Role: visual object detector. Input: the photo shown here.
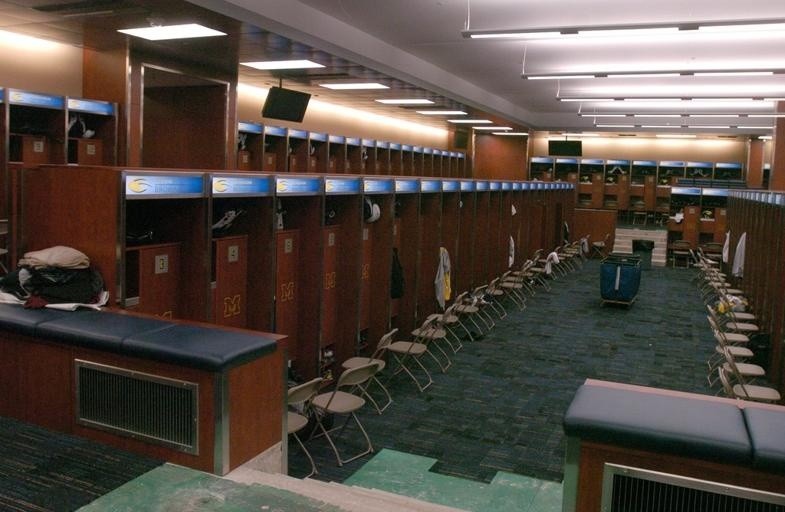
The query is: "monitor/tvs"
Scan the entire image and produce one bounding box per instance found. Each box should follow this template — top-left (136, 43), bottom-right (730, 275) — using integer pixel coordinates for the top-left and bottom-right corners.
top-left (549, 141), bottom-right (583, 157)
top-left (261, 87), bottom-right (312, 122)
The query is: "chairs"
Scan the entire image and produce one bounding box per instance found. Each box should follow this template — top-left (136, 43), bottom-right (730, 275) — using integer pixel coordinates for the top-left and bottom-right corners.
top-left (672, 237), bottom-right (781, 407)
